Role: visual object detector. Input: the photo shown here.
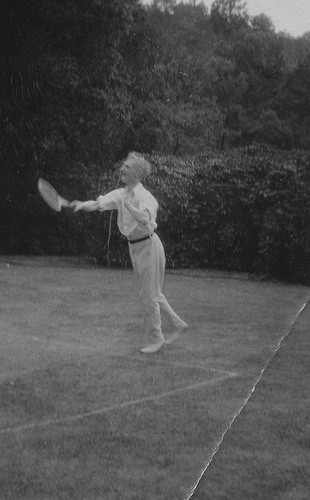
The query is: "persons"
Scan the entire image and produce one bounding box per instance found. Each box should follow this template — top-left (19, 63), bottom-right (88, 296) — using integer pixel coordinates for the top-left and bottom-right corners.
top-left (70, 151), bottom-right (189, 354)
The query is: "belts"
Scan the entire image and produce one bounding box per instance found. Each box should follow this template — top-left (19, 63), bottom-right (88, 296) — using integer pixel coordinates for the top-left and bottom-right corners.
top-left (128, 231), bottom-right (155, 244)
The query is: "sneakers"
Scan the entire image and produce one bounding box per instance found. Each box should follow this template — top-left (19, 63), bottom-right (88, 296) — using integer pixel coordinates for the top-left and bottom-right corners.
top-left (164, 322), bottom-right (189, 345)
top-left (140, 338), bottom-right (165, 353)
